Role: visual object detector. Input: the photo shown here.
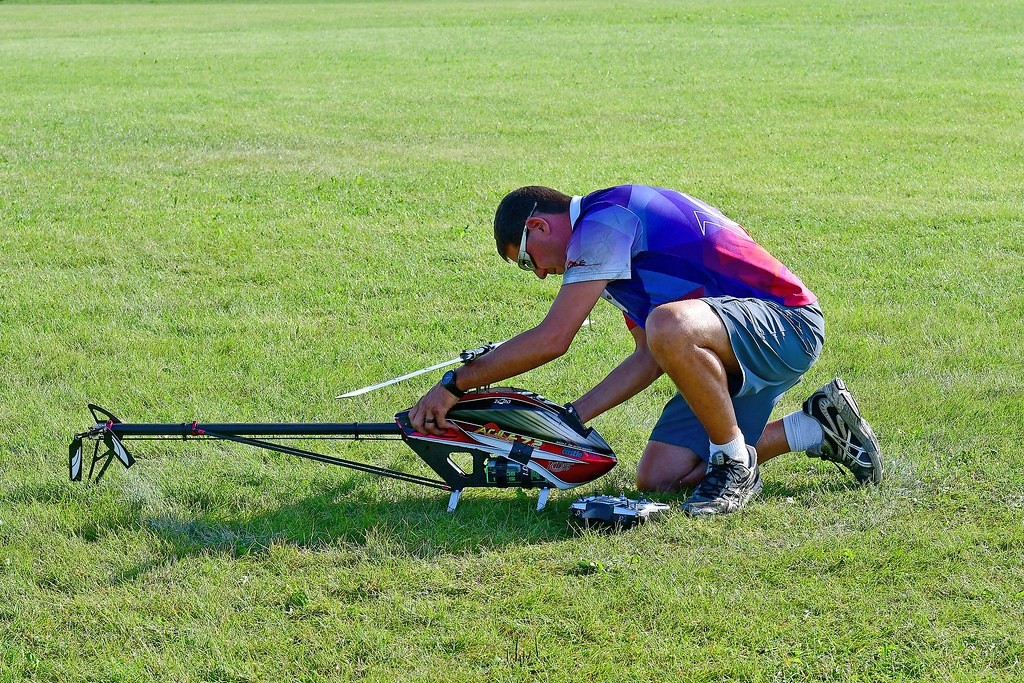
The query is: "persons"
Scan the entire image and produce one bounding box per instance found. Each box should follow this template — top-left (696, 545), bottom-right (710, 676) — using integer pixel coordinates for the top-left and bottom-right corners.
top-left (409, 185), bottom-right (884, 518)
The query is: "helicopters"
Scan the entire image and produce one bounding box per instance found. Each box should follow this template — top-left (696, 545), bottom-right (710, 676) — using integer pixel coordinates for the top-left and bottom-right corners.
top-left (69, 320), bottom-right (618, 513)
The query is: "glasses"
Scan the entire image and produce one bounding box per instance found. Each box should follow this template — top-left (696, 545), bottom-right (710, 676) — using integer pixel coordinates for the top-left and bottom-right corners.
top-left (517, 201), bottom-right (538, 271)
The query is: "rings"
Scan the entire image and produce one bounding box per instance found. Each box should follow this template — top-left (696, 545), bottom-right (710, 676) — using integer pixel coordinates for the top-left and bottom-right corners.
top-left (425, 418), bottom-right (435, 423)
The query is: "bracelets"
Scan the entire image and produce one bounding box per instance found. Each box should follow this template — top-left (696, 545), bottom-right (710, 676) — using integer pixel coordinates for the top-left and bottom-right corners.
top-left (563, 402), bottom-right (583, 425)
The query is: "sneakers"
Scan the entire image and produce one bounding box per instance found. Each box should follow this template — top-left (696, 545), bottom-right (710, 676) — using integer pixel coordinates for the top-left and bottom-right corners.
top-left (802, 376), bottom-right (884, 486)
top-left (681, 443), bottom-right (763, 518)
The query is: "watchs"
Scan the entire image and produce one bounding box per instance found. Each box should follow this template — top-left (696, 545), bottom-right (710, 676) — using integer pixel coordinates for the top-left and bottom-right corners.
top-left (441, 370), bottom-right (465, 398)
